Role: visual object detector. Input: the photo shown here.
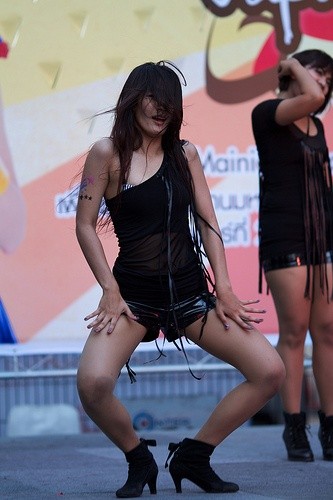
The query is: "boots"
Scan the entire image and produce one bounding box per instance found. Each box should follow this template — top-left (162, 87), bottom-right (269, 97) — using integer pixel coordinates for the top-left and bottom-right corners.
top-left (166, 437), bottom-right (239, 494)
top-left (116, 438), bottom-right (157, 497)
top-left (282, 410), bottom-right (316, 462)
top-left (318, 410), bottom-right (333, 461)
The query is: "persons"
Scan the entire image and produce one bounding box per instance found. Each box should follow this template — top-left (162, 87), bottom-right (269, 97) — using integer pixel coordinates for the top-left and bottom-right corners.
top-left (250, 49), bottom-right (333, 461)
top-left (74, 62), bottom-right (287, 497)
